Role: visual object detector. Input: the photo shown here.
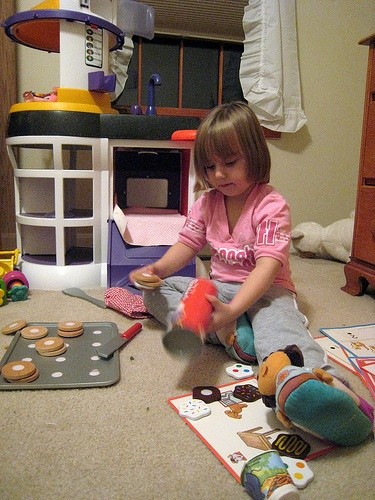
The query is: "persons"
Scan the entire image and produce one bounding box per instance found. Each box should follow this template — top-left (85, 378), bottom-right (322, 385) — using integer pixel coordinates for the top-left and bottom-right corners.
top-left (130, 102), bottom-right (375, 447)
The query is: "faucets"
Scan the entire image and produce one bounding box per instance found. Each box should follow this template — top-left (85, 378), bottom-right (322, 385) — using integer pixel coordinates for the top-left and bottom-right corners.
top-left (146, 73), bottom-right (163, 116)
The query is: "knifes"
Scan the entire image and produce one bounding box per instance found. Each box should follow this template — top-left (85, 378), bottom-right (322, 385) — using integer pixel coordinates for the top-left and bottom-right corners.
top-left (96, 323), bottom-right (142, 357)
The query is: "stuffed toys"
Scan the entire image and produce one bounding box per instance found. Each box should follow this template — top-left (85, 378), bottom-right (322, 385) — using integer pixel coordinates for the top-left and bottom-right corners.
top-left (258, 343), bottom-right (333, 429)
top-left (290, 212), bottom-right (355, 262)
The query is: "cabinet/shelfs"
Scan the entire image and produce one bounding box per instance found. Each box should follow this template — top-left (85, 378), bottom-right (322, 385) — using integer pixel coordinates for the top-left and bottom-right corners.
top-left (5, 102), bottom-right (201, 297)
top-left (340, 34), bottom-right (375, 300)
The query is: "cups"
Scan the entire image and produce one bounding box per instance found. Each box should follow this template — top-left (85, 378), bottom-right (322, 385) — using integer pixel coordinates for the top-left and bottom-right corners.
top-left (3, 270), bottom-right (30, 293)
